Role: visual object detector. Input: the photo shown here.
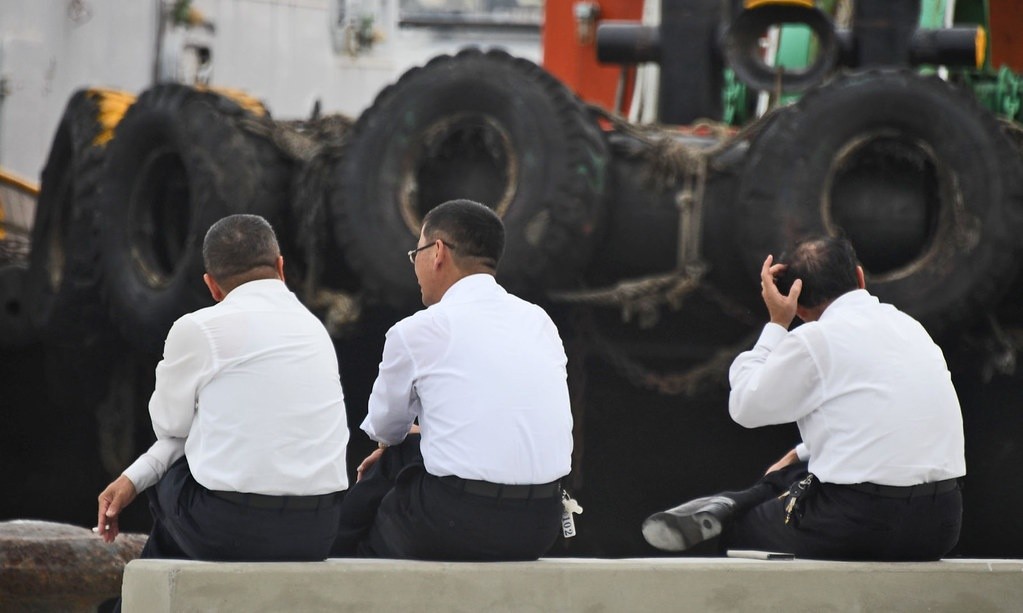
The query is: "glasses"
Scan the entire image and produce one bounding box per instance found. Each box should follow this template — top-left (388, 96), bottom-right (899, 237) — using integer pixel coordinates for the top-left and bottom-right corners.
top-left (407, 241), bottom-right (455, 264)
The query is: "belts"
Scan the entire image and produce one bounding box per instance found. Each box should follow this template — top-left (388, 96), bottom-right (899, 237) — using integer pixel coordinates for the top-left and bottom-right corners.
top-left (434, 475), bottom-right (562, 501)
top-left (202, 485), bottom-right (335, 513)
top-left (840, 477), bottom-right (960, 499)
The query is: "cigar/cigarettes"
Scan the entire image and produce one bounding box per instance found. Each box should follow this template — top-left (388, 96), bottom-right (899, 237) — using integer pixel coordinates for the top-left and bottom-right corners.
top-left (92, 525), bottom-right (109, 533)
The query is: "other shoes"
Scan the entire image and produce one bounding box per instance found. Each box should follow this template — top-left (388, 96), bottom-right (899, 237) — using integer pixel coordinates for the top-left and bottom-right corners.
top-left (96, 596), bottom-right (121, 613)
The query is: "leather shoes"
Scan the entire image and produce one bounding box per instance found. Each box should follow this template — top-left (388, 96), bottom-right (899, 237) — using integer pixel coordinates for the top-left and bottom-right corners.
top-left (640, 496), bottom-right (736, 552)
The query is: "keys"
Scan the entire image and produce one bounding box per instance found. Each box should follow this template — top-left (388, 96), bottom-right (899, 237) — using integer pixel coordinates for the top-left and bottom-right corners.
top-left (777, 480), bottom-right (808, 525)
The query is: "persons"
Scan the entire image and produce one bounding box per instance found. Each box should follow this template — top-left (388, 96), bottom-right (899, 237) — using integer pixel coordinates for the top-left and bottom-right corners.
top-left (642, 228), bottom-right (966, 561)
top-left (329, 199), bottom-right (575, 562)
top-left (97, 215), bottom-right (350, 613)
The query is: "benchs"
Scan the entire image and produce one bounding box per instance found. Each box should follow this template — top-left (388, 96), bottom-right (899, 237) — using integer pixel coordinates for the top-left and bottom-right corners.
top-left (119, 556), bottom-right (1023, 613)
